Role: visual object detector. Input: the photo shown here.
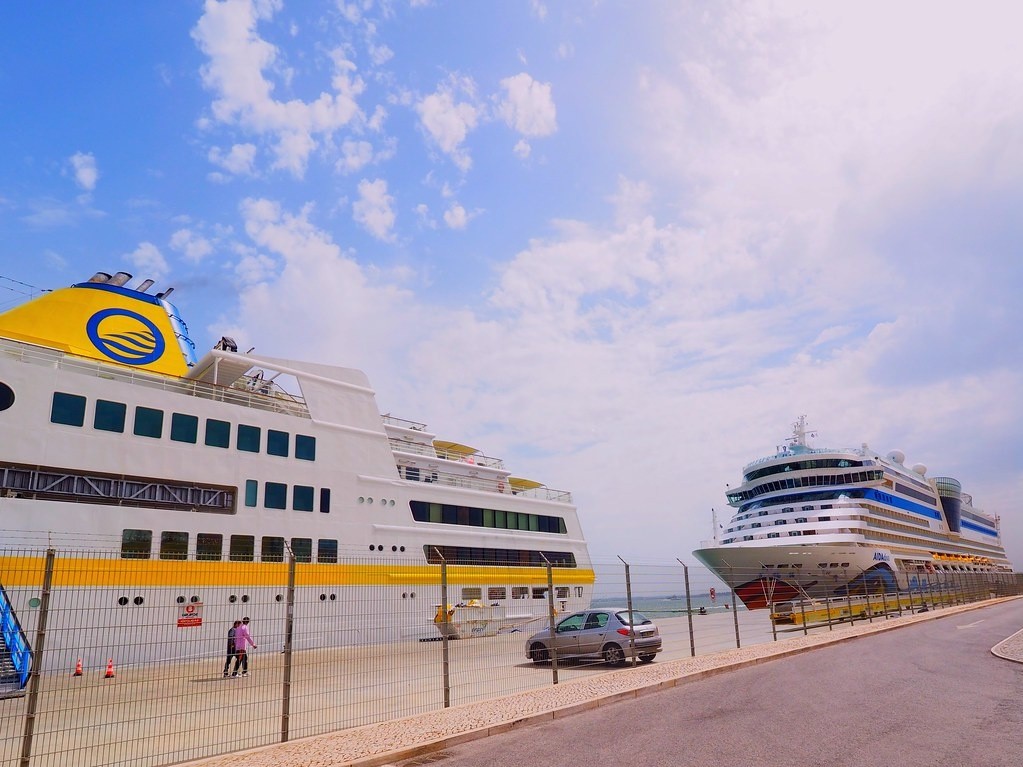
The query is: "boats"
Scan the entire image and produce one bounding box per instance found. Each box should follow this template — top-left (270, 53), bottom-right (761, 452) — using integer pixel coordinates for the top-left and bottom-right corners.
top-left (692, 414), bottom-right (1017, 614)
top-left (1, 271), bottom-right (596, 677)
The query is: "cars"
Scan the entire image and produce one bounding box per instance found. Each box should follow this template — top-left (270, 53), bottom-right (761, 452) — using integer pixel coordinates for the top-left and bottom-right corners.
top-left (525, 607), bottom-right (663, 668)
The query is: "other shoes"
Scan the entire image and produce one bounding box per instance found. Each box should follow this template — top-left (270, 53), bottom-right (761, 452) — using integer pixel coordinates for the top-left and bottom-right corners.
top-left (242, 672), bottom-right (250, 677)
top-left (231, 673), bottom-right (242, 679)
top-left (224, 675), bottom-right (230, 678)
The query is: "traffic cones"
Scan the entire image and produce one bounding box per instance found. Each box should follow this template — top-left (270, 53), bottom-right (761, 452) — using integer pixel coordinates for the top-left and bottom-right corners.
top-left (73, 658), bottom-right (84, 676)
top-left (105, 659), bottom-right (115, 678)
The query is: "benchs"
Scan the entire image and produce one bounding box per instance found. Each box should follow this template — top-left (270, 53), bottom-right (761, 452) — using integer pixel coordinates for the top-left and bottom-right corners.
top-left (822, 611), bottom-right (867, 623)
top-left (873, 606), bottom-right (902, 615)
top-left (906, 602), bottom-right (927, 609)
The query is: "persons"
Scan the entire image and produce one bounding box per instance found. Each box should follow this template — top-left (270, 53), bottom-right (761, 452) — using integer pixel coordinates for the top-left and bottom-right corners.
top-left (230, 617), bottom-right (258, 679)
top-left (699, 606), bottom-right (706, 613)
top-left (223, 620), bottom-right (241, 677)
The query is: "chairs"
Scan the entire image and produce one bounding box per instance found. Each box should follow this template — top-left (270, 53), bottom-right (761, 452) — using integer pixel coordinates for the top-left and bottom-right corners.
top-left (591, 617), bottom-right (601, 628)
